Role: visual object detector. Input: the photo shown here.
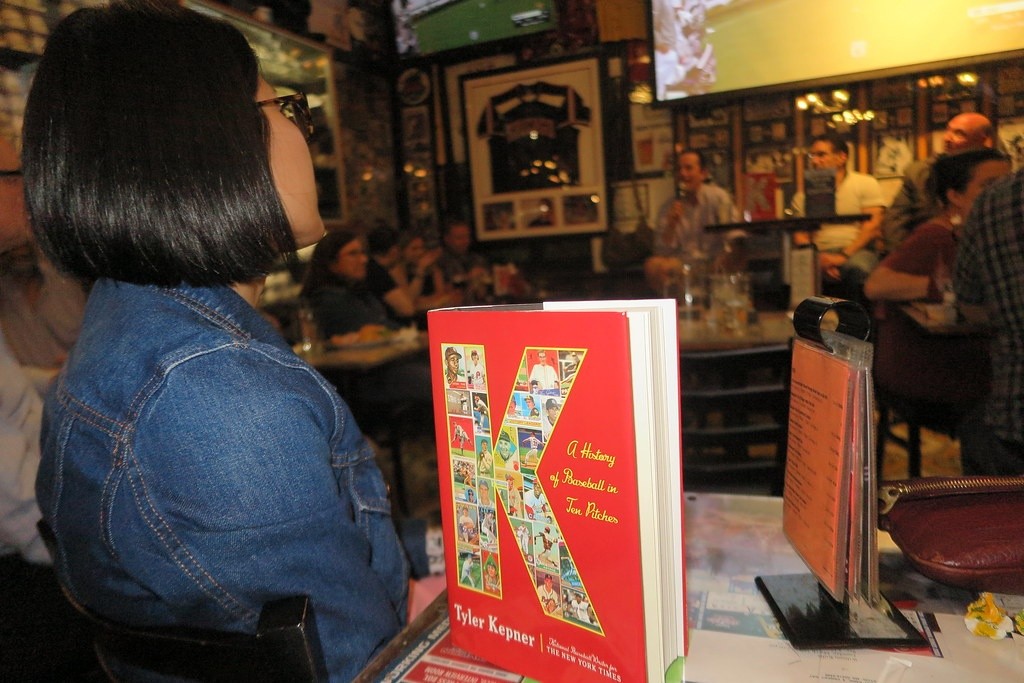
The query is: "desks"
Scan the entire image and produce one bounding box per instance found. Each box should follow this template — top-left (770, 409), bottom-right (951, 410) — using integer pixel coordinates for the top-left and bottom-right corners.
top-left (291, 331), bottom-right (430, 518)
top-left (672, 301), bottom-right (836, 458)
top-left (351, 489), bottom-right (1024, 683)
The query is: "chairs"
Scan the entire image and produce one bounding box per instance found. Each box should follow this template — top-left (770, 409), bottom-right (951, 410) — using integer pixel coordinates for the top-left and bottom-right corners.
top-left (673, 338), bottom-right (793, 495)
top-left (34, 518), bottom-right (329, 683)
top-left (839, 271), bottom-right (921, 483)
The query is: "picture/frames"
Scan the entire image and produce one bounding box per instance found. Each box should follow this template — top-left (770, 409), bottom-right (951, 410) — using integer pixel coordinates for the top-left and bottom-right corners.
top-left (458, 47), bottom-right (613, 247)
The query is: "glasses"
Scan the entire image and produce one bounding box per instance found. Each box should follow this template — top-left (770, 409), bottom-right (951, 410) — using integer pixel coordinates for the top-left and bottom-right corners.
top-left (255, 92), bottom-right (315, 146)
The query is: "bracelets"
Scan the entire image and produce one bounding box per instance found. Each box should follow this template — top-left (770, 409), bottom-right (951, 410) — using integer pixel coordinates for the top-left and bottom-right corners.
top-left (839, 250), bottom-right (848, 258)
top-left (927, 275), bottom-right (939, 301)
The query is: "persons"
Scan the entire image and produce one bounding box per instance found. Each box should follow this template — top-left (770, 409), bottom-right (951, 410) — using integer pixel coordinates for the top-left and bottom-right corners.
top-left (862, 113), bottom-right (1024, 480)
top-left (793, 133), bottom-right (882, 291)
top-left (646, 148), bottom-right (745, 294)
top-left (303, 220), bottom-right (490, 408)
top-left (1, 172), bottom-right (84, 573)
top-left (443, 346), bottom-right (598, 626)
top-left (21, 0), bottom-right (443, 683)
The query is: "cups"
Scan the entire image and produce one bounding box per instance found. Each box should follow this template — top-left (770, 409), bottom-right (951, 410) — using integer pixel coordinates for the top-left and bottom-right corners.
top-left (725, 300), bottom-right (745, 339)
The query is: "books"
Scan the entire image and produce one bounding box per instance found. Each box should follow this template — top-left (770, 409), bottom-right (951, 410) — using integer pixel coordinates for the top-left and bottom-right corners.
top-left (429, 297), bottom-right (689, 683)
top-left (740, 172), bottom-right (783, 220)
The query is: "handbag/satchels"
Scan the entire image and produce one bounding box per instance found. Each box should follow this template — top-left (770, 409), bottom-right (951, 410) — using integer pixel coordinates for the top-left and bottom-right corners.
top-left (876, 475), bottom-right (1024, 593)
